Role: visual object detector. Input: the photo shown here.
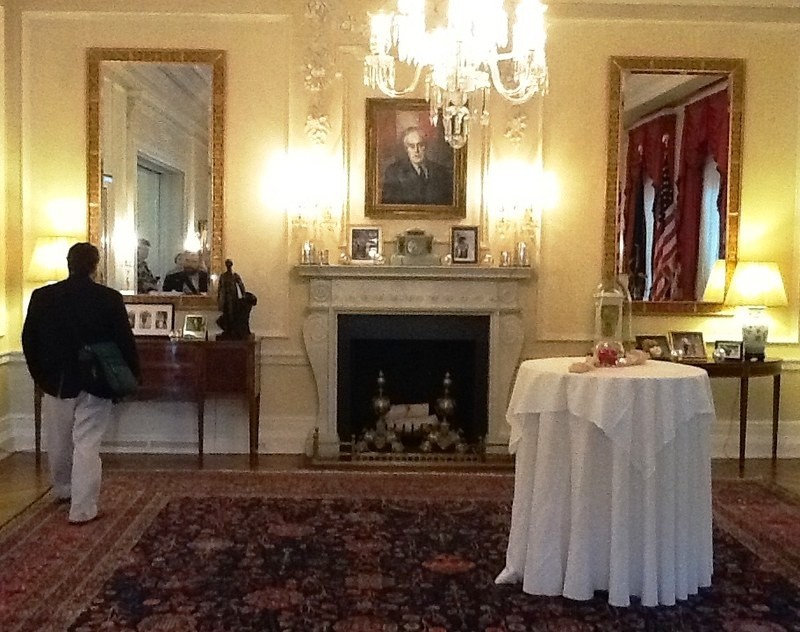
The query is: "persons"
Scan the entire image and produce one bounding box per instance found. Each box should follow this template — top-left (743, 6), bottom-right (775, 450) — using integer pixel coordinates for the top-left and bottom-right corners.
top-left (216, 258), bottom-right (245, 337)
top-left (21, 242), bottom-right (144, 525)
top-left (137, 238), bottom-right (208, 294)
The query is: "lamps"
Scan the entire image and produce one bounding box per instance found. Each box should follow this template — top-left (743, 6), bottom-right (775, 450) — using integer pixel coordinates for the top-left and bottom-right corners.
top-left (723, 260), bottom-right (788, 361)
top-left (363, 0), bottom-right (550, 146)
top-left (703, 256), bottom-right (725, 303)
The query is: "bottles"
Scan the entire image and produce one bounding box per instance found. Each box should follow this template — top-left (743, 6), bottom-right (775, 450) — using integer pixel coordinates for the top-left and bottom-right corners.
top-left (713, 346), bottom-right (726, 364)
top-left (595, 337), bottom-right (624, 366)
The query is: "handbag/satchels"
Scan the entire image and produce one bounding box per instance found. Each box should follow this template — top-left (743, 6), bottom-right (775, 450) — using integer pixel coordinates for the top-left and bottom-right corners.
top-left (84, 340), bottom-right (139, 395)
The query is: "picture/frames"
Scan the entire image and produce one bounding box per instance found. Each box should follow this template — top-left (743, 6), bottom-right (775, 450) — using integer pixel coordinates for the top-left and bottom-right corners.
top-left (126, 306), bottom-right (175, 334)
top-left (666, 328), bottom-right (708, 359)
top-left (348, 226), bottom-right (383, 265)
top-left (365, 97), bottom-right (466, 220)
top-left (449, 225), bottom-right (478, 264)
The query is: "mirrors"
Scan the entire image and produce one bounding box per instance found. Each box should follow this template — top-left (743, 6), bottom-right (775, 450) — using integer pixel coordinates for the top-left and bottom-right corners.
top-left (607, 53), bottom-right (746, 321)
top-left (85, 46), bottom-right (227, 309)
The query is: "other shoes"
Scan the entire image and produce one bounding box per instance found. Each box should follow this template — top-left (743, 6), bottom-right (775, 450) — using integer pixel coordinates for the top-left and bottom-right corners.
top-left (95, 511), bottom-right (106, 518)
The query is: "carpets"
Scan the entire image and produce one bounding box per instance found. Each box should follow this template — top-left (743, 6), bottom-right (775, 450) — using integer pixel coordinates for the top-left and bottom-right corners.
top-left (0, 470), bottom-right (799, 632)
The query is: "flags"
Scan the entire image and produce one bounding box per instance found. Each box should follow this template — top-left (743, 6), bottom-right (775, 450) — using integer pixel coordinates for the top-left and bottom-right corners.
top-left (623, 134), bottom-right (681, 300)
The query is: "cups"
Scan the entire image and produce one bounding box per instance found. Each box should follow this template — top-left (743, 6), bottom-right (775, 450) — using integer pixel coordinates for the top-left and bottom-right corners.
top-left (670, 349), bottom-right (683, 361)
top-left (516, 242), bottom-right (526, 267)
top-left (500, 251), bottom-right (512, 266)
top-left (302, 243), bottom-right (314, 264)
top-left (318, 250), bottom-right (329, 265)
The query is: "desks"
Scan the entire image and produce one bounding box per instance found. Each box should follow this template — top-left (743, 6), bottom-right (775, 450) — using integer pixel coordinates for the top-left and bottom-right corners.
top-left (658, 359), bottom-right (783, 469)
top-left (33, 334), bottom-right (265, 475)
top-left (495, 357), bottom-right (718, 606)
